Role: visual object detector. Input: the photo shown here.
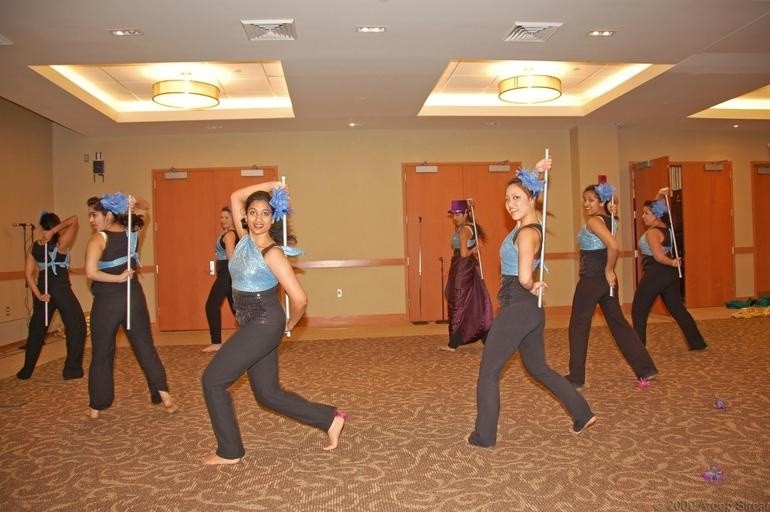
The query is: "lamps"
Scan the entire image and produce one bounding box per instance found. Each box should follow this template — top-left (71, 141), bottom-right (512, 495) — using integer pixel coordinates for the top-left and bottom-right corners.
top-left (149, 80), bottom-right (224, 110)
top-left (497, 77), bottom-right (564, 106)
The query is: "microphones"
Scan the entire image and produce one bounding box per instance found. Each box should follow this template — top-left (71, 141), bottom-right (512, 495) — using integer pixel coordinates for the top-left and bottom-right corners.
top-left (13, 223), bottom-right (32, 226)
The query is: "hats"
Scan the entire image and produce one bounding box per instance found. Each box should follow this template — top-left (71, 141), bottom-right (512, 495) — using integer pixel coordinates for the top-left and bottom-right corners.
top-left (448, 199), bottom-right (473, 214)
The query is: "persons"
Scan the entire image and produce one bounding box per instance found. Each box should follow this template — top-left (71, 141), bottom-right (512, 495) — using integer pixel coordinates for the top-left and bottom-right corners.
top-left (14, 180), bottom-right (346, 466)
top-left (439, 157), bottom-right (708, 450)
